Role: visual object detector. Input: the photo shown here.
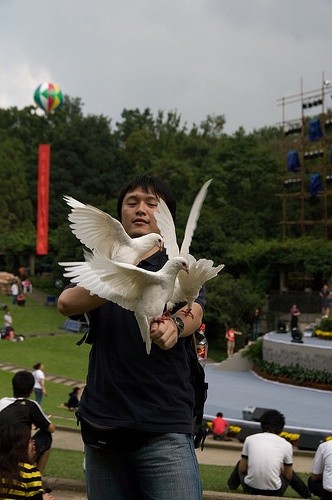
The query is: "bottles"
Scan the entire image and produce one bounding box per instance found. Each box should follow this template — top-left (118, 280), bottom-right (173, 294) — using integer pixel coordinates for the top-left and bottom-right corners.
top-left (196, 323), bottom-right (208, 368)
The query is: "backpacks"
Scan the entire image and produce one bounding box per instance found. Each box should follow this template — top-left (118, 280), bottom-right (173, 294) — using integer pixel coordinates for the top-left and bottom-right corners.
top-left (173, 300), bottom-right (208, 451)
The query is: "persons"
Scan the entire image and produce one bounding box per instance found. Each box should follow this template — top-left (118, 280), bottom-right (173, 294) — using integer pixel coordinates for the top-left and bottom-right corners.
top-left (61, 386), bottom-right (80, 411)
top-left (0, 371), bottom-right (55, 494)
top-left (225, 326), bottom-right (243, 357)
top-left (319, 285), bottom-right (332, 318)
top-left (290, 304), bottom-right (301, 329)
top-left (32, 363), bottom-right (47, 405)
top-left (227, 409), bottom-right (313, 499)
top-left (212, 412), bottom-right (232, 442)
top-left (250, 307), bottom-right (260, 340)
top-left (10, 280), bottom-right (20, 304)
top-left (0, 423), bottom-right (56, 500)
top-left (4, 309), bottom-right (13, 337)
top-left (307, 439), bottom-right (332, 500)
top-left (57, 176), bottom-right (207, 500)
top-left (22, 278), bottom-right (32, 294)
top-left (16, 293), bottom-right (27, 306)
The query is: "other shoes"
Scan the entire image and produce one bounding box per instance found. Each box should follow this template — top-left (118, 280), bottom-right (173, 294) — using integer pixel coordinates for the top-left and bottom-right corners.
top-left (227, 485), bottom-right (238, 492)
top-left (41, 482), bottom-right (52, 493)
top-left (308, 492), bottom-right (314, 499)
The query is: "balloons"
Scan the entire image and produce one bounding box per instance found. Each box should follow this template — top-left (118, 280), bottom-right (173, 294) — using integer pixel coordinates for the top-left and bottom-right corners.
top-left (33, 82), bottom-right (64, 112)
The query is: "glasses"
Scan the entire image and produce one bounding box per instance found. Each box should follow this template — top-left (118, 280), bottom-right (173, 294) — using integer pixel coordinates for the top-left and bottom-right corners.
top-left (30, 438), bottom-right (35, 444)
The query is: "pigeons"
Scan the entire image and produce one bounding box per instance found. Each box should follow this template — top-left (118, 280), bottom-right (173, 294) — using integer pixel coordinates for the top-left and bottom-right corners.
top-left (58, 178), bottom-right (225, 354)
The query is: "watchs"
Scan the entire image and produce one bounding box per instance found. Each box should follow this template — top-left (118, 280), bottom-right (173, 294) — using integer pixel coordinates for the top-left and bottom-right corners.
top-left (170, 314), bottom-right (185, 339)
